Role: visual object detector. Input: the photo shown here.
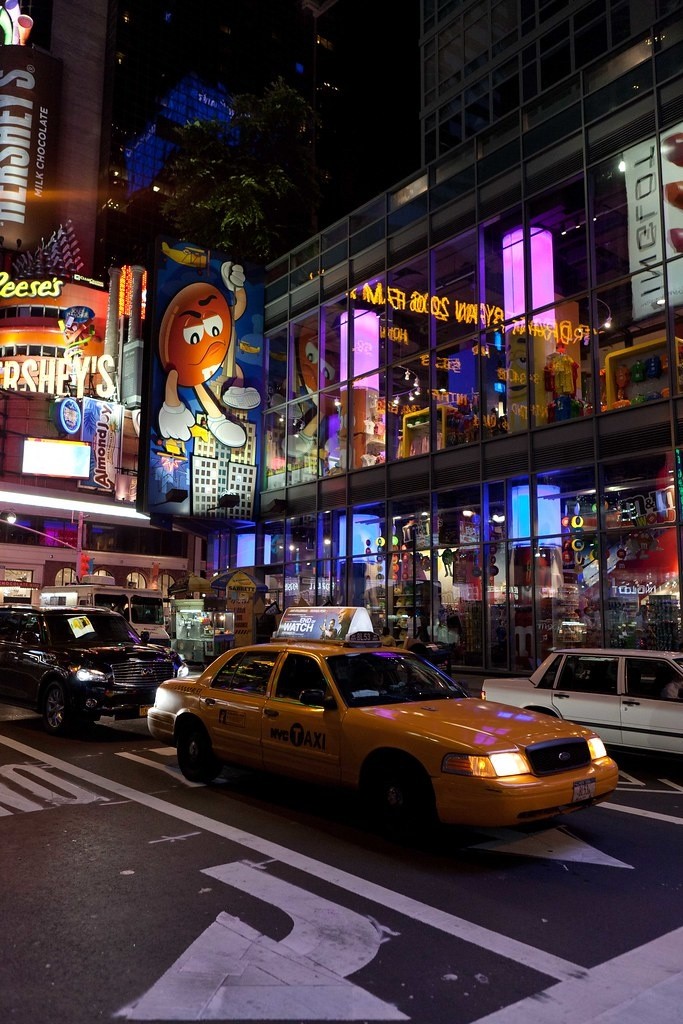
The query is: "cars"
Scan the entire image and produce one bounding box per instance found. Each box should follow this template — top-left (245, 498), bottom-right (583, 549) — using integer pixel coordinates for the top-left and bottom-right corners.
top-left (146, 606), bottom-right (618, 853)
top-left (481, 647), bottom-right (683, 758)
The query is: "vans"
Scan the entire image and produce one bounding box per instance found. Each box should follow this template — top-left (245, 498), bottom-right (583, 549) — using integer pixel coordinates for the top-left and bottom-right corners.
top-left (39, 575), bottom-right (171, 649)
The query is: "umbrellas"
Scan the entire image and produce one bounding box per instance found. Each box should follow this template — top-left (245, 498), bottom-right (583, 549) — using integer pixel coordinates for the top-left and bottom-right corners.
top-left (166, 572), bottom-right (216, 598)
top-left (210, 570), bottom-right (268, 612)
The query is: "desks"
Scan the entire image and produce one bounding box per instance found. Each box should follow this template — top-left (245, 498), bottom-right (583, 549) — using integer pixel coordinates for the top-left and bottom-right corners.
top-left (402, 405), bottom-right (454, 457)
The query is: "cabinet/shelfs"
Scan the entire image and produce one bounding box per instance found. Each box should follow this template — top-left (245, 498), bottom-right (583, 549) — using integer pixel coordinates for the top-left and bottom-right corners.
top-left (371, 582), bottom-right (678, 650)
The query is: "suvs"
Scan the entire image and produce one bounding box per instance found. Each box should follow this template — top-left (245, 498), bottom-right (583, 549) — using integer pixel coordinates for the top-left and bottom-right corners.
top-left (0, 602), bottom-right (190, 738)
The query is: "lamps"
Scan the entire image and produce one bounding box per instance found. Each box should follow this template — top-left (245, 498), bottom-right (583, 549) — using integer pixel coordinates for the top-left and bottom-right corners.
top-left (256, 500), bottom-right (284, 517)
top-left (205, 494), bottom-right (240, 514)
top-left (148, 489), bottom-right (188, 510)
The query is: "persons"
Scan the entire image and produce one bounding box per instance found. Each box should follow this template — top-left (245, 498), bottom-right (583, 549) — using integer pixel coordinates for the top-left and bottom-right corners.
top-left (569, 603), bottom-right (658, 648)
top-left (320, 606), bottom-right (353, 640)
top-left (660, 662), bottom-right (683, 699)
top-left (208, 614), bottom-right (225, 629)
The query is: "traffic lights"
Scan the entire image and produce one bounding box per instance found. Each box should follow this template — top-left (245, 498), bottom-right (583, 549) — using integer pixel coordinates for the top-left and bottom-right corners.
top-left (78, 553), bottom-right (90, 579)
